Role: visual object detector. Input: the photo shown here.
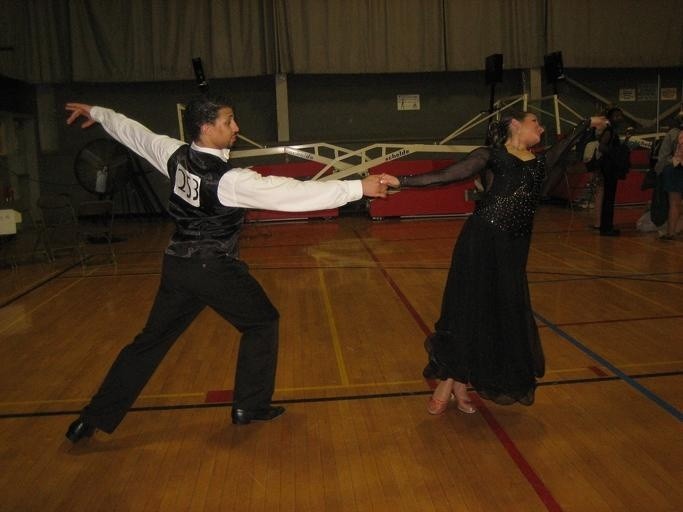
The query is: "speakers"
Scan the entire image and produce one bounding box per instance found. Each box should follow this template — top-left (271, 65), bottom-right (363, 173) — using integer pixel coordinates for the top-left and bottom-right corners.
top-left (483, 53), bottom-right (504, 83)
top-left (543, 51), bottom-right (564, 85)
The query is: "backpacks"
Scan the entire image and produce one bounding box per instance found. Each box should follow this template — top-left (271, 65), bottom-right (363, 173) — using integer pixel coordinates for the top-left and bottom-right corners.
top-left (582, 128), bottom-right (613, 173)
top-left (671, 125), bottom-right (683, 168)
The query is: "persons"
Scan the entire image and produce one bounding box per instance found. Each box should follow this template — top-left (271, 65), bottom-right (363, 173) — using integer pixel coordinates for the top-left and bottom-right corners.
top-left (377, 109), bottom-right (610, 415)
top-left (570, 107), bottom-right (683, 240)
top-left (64, 96), bottom-right (389, 444)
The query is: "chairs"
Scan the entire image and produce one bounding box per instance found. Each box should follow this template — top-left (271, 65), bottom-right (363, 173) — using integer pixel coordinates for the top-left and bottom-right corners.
top-left (0, 192), bottom-right (122, 276)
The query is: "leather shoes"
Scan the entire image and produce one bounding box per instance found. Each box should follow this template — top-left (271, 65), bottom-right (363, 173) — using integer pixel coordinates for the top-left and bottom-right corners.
top-left (64, 417), bottom-right (97, 444)
top-left (452, 388), bottom-right (479, 415)
top-left (229, 403), bottom-right (286, 425)
top-left (426, 384), bottom-right (451, 415)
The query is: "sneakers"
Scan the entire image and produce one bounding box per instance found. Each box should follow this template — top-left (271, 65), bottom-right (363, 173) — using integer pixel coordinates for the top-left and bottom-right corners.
top-left (655, 233), bottom-right (677, 242)
top-left (599, 226), bottom-right (622, 236)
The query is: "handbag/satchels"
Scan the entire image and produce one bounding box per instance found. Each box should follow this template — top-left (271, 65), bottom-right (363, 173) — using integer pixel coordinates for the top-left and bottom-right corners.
top-left (648, 173), bottom-right (671, 229)
